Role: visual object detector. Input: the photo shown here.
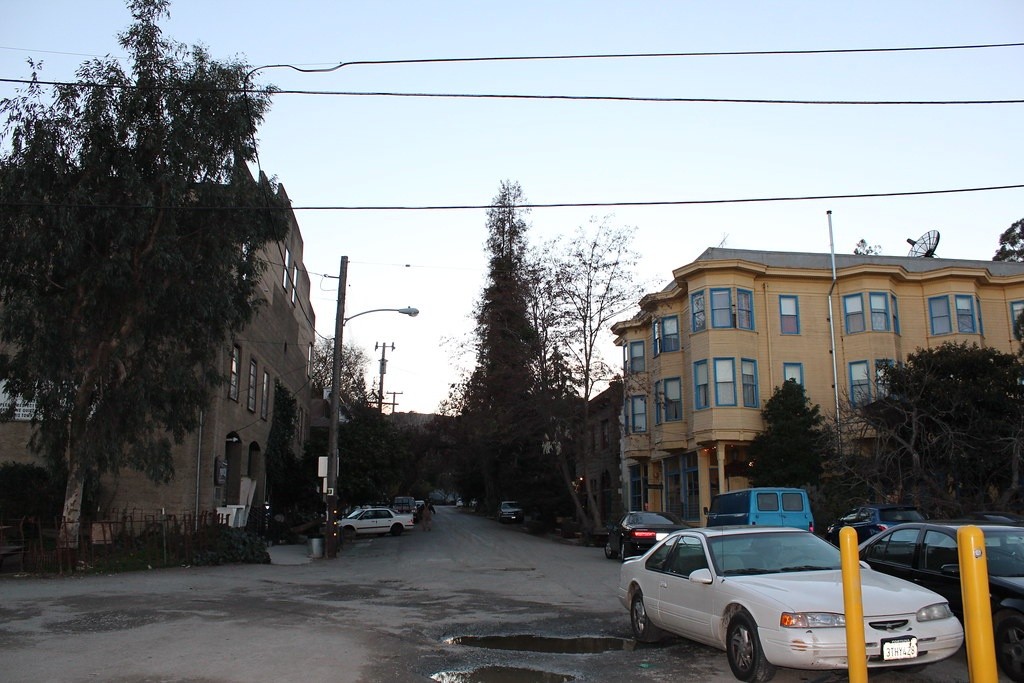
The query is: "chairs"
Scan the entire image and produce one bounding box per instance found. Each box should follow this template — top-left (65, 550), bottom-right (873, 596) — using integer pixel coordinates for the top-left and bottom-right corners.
top-left (718, 554), bottom-right (743, 570)
top-left (777, 548), bottom-right (803, 567)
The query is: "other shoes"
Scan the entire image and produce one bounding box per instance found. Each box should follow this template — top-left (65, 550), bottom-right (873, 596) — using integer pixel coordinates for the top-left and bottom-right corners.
top-left (423, 528), bottom-right (427, 532)
top-left (428, 528), bottom-right (431, 532)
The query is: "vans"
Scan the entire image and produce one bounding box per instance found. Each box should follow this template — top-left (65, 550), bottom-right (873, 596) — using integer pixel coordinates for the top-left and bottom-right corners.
top-left (702, 486), bottom-right (814, 533)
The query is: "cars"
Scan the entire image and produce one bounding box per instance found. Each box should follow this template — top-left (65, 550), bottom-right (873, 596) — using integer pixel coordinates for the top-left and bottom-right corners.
top-left (604, 510), bottom-right (694, 564)
top-left (336, 507), bottom-right (415, 537)
top-left (823, 502), bottom-right (927, 550)
top-left (855, 519), bottom-right (1024, 683)
top-left (391, 496), bottom-right (418, 523)
top-left (615, 524), bottom-right (965, 682)
top-left (497, 500), bottom-right (525, 523)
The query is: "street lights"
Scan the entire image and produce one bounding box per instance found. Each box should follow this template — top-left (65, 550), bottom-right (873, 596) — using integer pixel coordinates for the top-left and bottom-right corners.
top-left (325, 255), bottom-right (419, 559)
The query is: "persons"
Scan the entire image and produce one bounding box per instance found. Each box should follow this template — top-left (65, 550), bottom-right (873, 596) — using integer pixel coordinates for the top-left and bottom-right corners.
top-left (417, 499), bottom-right (436, 533)
